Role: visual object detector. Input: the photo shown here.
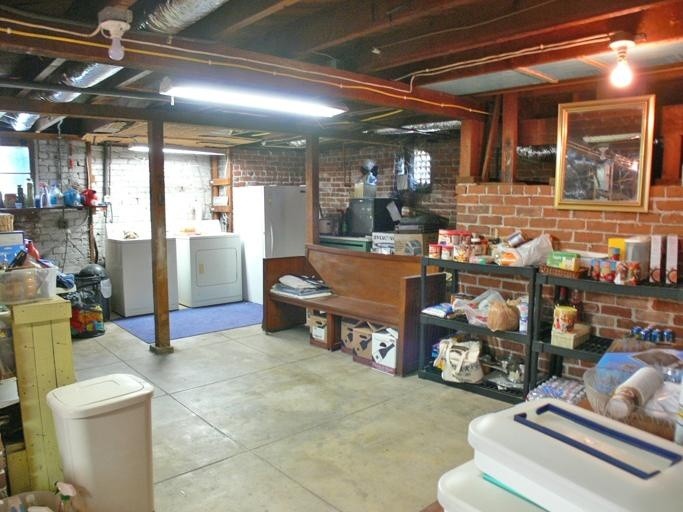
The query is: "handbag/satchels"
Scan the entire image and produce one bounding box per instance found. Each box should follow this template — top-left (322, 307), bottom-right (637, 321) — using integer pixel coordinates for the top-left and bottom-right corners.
top-left (439, 339), bottom-right (485, 383)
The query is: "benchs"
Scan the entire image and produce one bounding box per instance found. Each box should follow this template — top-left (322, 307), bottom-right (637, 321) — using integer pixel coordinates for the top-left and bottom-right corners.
top-left (262, 244), bottom-right (444, 377)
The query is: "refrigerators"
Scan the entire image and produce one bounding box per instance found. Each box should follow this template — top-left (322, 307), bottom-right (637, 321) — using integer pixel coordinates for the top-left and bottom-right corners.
top-left (231, 184), bottom-right (306, 307)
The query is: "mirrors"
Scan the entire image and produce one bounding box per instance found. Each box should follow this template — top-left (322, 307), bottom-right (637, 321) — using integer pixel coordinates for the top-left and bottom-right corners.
top-left (553, 94), bottom-right (656, 215)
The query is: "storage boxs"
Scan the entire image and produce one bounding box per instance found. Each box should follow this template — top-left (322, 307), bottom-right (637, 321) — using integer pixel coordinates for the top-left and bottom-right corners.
top-left (0, 259), bottom-right (59, 305)
top-left (309, 308), bottom-right (399, 376)
top-left (437, 398), bottom-right (682, 512)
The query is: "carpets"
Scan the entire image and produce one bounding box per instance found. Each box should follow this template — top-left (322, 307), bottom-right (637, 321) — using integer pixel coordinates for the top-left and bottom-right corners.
top-left (111, 301), bottom-right (263, 344)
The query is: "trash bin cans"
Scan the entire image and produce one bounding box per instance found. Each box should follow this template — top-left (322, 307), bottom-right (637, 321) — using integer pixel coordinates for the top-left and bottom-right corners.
top-left (46, 373), bottom-right (154, 512)
top-left (75, 264), bottom-right (110, 322)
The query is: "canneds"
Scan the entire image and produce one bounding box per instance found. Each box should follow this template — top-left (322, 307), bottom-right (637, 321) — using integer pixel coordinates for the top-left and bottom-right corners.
top-left (470, 237), bottom-right (482, 256)
top-left (631, 325), bottom-right (674, 343)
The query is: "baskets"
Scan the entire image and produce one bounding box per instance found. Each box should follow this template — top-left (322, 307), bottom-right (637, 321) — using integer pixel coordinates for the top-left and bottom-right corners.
top-left (551, 322), bottom-right (591, 350)
top-left (537, 261), bottom-right (589, 280)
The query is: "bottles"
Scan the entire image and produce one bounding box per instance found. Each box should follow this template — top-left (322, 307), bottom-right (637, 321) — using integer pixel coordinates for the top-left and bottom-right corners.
top-left (604, 363), bottom-right (664, 423)
top-left (16, 184), bottom-right (24, 208)
top-left (25, 177), bottom-right (34, 208)
top-left (34, 195), bottom-right (41, 208)
top-left (552, 305), bottom-right (578, 333)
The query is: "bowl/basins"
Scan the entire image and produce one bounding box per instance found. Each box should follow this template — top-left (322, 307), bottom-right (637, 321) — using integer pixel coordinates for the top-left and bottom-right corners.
top-left (582, 362), bottom-right (683, 441)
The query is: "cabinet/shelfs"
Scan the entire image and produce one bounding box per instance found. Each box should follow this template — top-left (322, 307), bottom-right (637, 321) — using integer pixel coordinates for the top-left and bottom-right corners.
top-left (420, 255), bottom-right (682, 405)
top-left (0, 294), bottom-right (76, 497)
